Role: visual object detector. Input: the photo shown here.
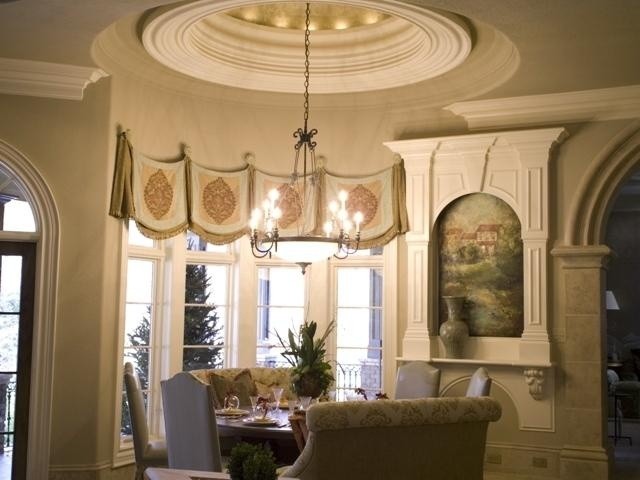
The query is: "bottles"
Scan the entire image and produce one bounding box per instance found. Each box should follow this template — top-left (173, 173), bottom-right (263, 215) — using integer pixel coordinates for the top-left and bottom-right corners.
top-left (223, 390), bottom-right (240, 409)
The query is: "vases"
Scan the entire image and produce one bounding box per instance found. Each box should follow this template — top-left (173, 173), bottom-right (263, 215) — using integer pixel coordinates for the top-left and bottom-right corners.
top-left (440, 295), bottom-right (470, 360)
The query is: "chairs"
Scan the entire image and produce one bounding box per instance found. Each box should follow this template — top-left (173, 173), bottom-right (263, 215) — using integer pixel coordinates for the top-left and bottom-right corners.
top-left (123, 362), bottom-right (502, 480)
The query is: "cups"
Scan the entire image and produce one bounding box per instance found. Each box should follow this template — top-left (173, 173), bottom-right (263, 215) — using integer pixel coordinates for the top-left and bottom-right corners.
top-left (298, 396), bottom-right (312, 409)
top-left (287, 397), bottom-right (298, 417)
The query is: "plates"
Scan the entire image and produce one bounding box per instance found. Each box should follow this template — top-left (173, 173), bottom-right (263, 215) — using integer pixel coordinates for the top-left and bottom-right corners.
top-left (243, 416), bottom-right (279, 426)
top-left (215, 408), bottom-right (249, 416)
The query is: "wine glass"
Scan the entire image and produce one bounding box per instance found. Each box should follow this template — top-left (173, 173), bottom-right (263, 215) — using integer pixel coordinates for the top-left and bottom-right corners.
top-left (270, 387), bottom-right (284, 413)
top-left (248, 395), bottom-right (259, 418)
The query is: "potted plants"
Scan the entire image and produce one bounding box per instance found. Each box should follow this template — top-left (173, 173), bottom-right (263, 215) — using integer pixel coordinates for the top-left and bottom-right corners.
top-left (273, 318), bottom-right (339, 399)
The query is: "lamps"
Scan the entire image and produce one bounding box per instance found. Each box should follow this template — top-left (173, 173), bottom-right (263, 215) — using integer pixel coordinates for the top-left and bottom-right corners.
top-left (606, 290), bottom-right (620, 310)
top-left (248, 2), bottom-right (364, 276)
